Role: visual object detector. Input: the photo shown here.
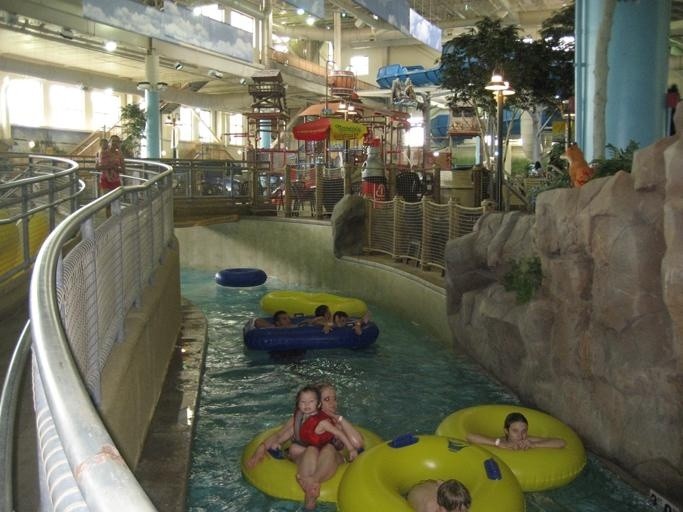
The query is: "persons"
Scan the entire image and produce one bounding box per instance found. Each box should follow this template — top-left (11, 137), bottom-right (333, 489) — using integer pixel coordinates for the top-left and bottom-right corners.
top-left (466, 411), bottom-right (566, 449)
top-left (99, 139), bottom-right (121, 182)
top-left (407, 479), bottom-right (472, 512)
top-left (94, 135), bottom-right (125, 217)
top-left (251, 383), bottom-right (364, 512)
top-left (529, 162), bottom-right (545, 176)
top-left (255, 305), bottom-right (370, 336)
top-left (666, 84), bottom-right (680, 136)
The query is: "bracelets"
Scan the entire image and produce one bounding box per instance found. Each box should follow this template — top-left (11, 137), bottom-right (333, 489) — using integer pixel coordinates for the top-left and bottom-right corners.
top-left (495, 438), bottom-right (500, 446)
